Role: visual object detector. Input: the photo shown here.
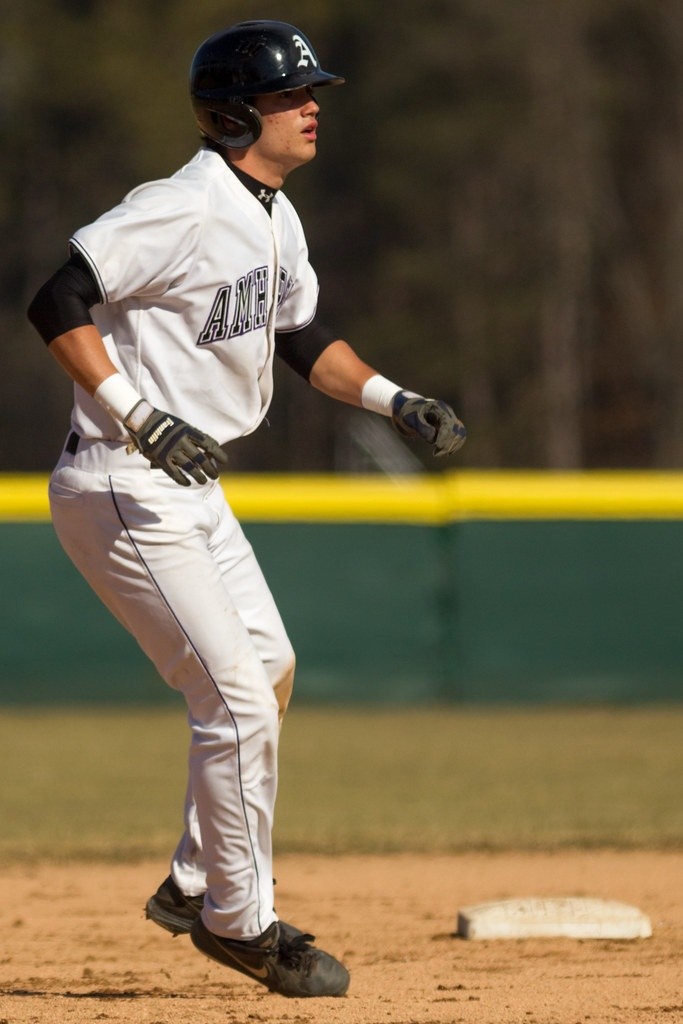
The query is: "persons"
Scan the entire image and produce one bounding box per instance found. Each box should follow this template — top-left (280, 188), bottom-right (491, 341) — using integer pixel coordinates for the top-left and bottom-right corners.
top-left (26, 20), bottom-right (468, 998)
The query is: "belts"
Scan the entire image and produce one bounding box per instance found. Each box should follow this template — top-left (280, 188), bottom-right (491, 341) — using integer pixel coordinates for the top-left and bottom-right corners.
top-left (65, 431), bottom-right (83, 455)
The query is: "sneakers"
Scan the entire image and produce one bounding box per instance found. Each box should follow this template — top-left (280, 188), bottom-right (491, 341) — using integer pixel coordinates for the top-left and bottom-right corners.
top-left (189, 906), bottom-right (352, 999)
top-left (143, 873), bottom-right (211, 935)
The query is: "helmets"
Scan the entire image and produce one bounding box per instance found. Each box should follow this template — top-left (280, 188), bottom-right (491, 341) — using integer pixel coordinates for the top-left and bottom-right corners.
top-left (188, 18), bottom-right (348, 152)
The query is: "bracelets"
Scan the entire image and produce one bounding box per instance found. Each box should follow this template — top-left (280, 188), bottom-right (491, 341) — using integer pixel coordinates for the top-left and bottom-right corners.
top-left (361, 375), bottom-right (404, 417)
top-left (92, 373), bottom-right (143, 422)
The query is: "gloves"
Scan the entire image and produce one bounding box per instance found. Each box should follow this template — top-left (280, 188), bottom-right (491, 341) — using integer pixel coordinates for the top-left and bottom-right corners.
top-left (392, 386), bottom-right (465, 460)
top-left (122, 403), bottom-right (229, 488)
